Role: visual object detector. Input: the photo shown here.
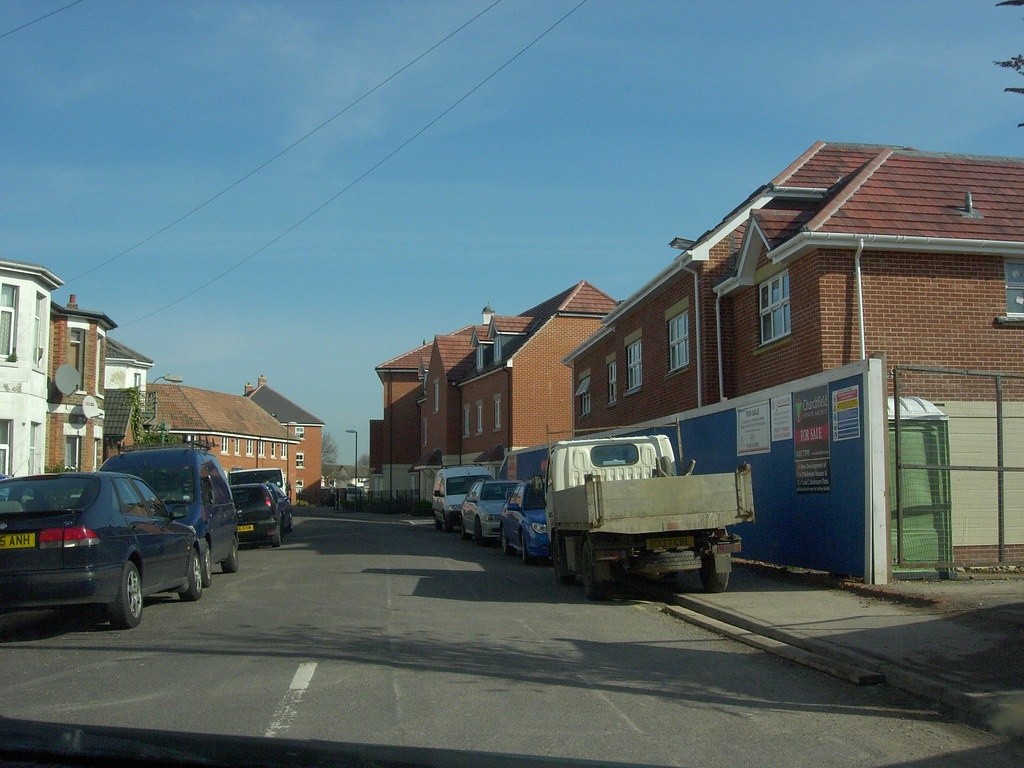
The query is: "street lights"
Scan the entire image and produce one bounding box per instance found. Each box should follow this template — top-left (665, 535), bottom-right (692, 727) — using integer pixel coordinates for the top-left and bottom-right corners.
top-left (345, 429), bottom-right (358, 513)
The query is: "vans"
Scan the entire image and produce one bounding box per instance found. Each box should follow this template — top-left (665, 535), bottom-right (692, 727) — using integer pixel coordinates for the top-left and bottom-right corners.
top-left (431, 467), bottom-right (495, 532)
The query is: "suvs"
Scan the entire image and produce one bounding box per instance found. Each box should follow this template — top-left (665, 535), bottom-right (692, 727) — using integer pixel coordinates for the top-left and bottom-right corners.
top-left (96, 440), bottom-right (239, 573)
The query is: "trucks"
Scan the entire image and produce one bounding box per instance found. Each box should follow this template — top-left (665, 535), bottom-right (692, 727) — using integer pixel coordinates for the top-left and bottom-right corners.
top-left (545, 434), bottom-right (756, 601)
top-left (228, 467), bottom-right (287, 497)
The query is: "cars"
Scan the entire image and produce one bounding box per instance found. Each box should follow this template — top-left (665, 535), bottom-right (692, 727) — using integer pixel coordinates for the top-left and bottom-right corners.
top-left (0, 471), bottom-right (202, 629)
top-left (500, 481), bottom-right (548, 563)
top-left (462, 478), bottom-right (523, 545)
top-left (229, 483), bottom-right (294, 547)
